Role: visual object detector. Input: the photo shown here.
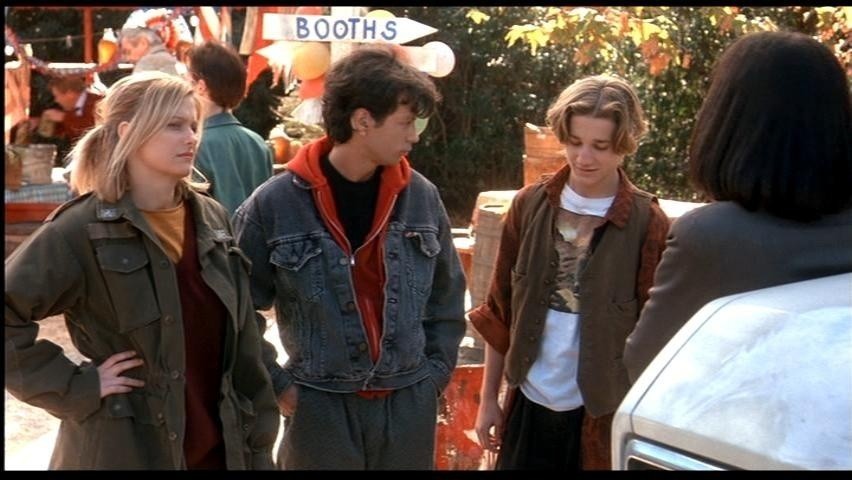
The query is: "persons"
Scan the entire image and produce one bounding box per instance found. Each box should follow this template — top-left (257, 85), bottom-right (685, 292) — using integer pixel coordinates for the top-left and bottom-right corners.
top-left (467, 76), bottom-right (672, 471)
top-left (622, 32), bottom-right (852, 382)
